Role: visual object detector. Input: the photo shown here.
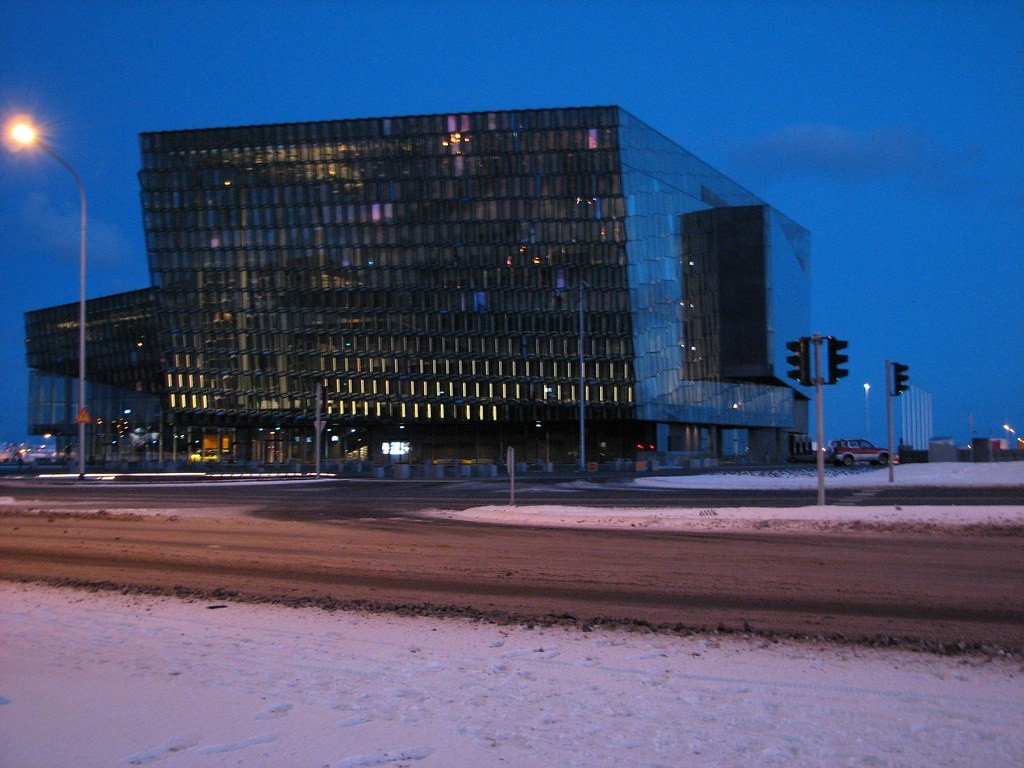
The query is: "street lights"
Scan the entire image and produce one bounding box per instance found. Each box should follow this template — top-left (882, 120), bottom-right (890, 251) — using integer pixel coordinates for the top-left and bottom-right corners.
top-left (553, 267), bottom-right (594, 469)
top-left (13, 123), bottom-right (88, 481)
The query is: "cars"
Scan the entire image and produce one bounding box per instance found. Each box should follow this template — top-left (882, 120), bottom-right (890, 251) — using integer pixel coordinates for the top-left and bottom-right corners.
top-left (828, 438), bottom-right (891, 467)
top-left (0, 444), bottom-right (50, 465)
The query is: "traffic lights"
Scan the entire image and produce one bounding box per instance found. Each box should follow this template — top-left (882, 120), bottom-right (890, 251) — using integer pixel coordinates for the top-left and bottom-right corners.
top-left (895, 362), bottom-right (911, 394)
top-left (827, 336), bottom-right (850, 382)
top-left (784, 336), bottom-right (811, 388)
